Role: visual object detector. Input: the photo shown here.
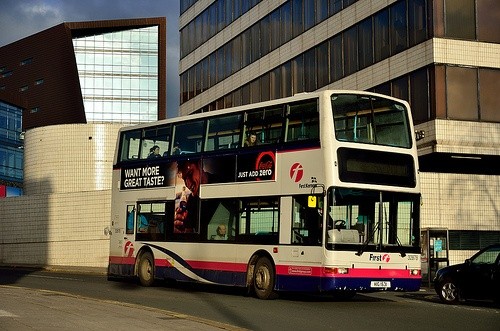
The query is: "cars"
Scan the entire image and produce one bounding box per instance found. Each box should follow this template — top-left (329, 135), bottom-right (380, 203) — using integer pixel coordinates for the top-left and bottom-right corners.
top-left (432, 243), bottom-right (500, 305)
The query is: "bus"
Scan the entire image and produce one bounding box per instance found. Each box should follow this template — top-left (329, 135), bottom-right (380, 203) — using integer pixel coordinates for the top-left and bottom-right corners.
top-left (106, 90), bottom-right (422, 299)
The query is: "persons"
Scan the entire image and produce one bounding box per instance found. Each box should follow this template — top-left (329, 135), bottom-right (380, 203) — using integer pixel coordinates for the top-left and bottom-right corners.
top-left (148, 146), bottom-right (162, 157)
top-left (244, 135), bottom-right (258, 148)
top-left (128, 204), bottom-right (148, 232)
top-left (175, 155), bottom-right (217, 231)
top-left (214, 224), bottom-right (229, 240)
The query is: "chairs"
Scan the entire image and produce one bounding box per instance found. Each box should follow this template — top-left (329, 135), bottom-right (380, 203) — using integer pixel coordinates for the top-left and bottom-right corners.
top-left (130, 133), bottom-right (311, 243)
